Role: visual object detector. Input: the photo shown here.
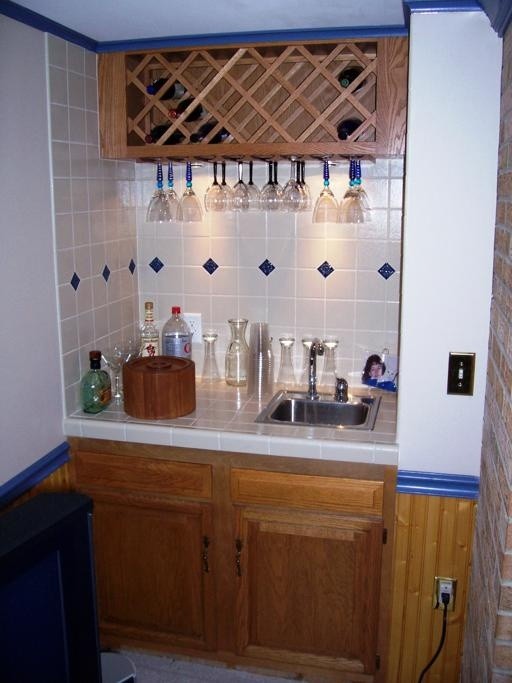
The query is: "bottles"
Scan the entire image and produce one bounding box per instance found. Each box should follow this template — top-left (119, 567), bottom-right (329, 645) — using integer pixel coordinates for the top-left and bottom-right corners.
top-left (140, 300), bottom-right (194, 361)
top-left (202, 317), bottom-right (249, 387)
top-left (336, 64), bottom-right (371, 143)
top-left (277, 337), bottom-right (339, 397)
top-left (80, 351), bottom-right (112, 413)
top-left (144, 78), bottom-right (232, 148)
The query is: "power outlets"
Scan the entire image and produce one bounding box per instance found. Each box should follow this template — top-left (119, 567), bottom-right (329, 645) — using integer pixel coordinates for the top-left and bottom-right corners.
top-left (183, 312), bottom-right (203, 345)
top-left (432, 576), bottom-right (457, 612)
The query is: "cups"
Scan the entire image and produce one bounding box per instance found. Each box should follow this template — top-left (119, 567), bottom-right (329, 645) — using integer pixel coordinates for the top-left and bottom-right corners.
top-left (248, 321), bottom-right (275, 402)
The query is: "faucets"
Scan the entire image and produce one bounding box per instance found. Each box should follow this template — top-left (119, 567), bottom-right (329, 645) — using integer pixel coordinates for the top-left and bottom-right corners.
top-left (307, 342), bottom-right (325, 400)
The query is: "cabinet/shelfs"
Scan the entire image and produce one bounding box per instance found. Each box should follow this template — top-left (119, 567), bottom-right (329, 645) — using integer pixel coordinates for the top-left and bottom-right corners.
top-left (69, 436), bottom-right (386, 675)
top-left (97, 38), bottom-right (409, 164)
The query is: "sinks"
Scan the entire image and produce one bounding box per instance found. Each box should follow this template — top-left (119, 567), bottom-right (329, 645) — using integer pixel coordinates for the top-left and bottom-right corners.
top-left (253, 389), bottom-right (381, 429)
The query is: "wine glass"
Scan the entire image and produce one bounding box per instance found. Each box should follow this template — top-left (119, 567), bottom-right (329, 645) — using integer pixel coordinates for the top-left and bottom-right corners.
top-left (145, 155), bottom-right (377, 224)
top-left (96, 340), bottom-right (145, 405)
